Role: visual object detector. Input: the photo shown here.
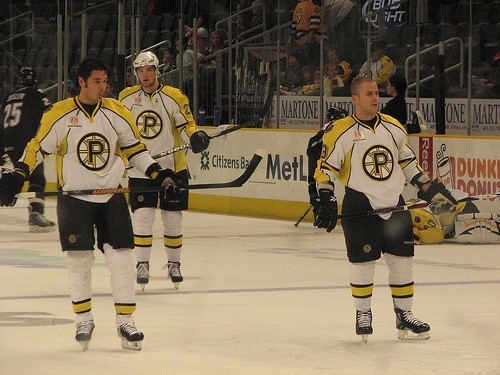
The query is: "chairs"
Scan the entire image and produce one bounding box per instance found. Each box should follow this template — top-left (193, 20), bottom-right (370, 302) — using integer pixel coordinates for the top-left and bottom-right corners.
top-left (0, 0), bottom-right (295, 104)
top-left (351, 0), bottom-right (500, 76)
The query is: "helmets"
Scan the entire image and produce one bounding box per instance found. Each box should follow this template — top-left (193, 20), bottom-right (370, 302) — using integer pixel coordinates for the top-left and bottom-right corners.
top-left (328, 107), bottom-right (348, 121)
top-left (132, 51), bottom-right (159, 71)
top-left (20, 68), bottom-right (37, 84)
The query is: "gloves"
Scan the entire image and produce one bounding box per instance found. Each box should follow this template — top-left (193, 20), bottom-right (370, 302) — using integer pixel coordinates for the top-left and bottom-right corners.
top-left (153, 168), bottom-right (185, 202)
top-left (0, 171), bottom-right (24, 207)
top-left (190, 131), bottom-right (209, 154)
top-left (418, 178), bottom-right (458, 215)
top-left (313, 188), bottom-right (338, 233)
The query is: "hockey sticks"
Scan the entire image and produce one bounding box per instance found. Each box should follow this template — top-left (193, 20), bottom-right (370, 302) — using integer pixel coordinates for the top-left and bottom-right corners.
top-left (125, 79), bottom-right (273, 171)
top-left (294, 204), bottom-right (313, 227)
top-left (337, 191), bottom-right (500, 220)
top-left (13, 149), bottom-right (266, 198)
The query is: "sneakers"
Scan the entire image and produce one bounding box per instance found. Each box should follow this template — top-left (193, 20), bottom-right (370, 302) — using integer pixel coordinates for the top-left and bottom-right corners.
top-left (75, 320), bottom-right (94, 351)
top-left (163, 261), bottom-right (183, 290)
top-left (392, 298), bottom-right (430, 340)
top-left (136, 261), bottom-right (150, 290)
top-left (117, 323), bottom-right (144, 350)
top-left (27, 206), bottom-right (55, 232)
top-left (356, 308), bottom-right (372, 343)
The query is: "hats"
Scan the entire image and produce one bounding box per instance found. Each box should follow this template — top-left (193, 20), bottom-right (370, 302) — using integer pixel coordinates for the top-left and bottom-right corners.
top-left (370, 41), bottom-right (380, 52)
top-left (197, 27), bottom-right (208, 38)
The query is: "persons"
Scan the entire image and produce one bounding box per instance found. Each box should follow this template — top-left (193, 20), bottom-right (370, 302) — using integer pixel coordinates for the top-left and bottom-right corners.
top-left (119, 51), bottom-right (208, 291)
top-left (306, 107), bottom-right (349, 218)
top-left (315, 76), bottom-right (457, 343)
top-left (0, 66), bottom-right (57, 233)
top-left (64, 0), bottom-right (500, 134)
top-left (0, 58), bottom-right (187, 351)
